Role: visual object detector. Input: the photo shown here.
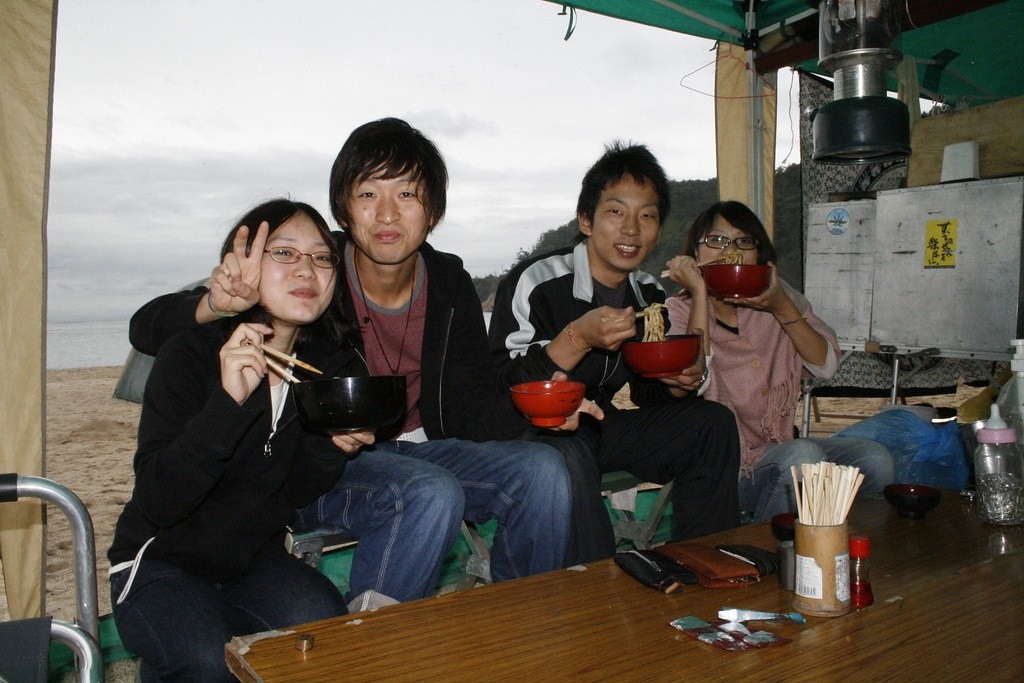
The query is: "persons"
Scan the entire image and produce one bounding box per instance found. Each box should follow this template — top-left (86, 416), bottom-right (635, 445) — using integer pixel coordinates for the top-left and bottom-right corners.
top-left (106, 199), bottom-right (378, 683)
top-left (129, 118), bottom-right (573, 614)
top-left (665, 201), bottom-right (895, 524)
top-left (489, 142), bottom-right (740, 569)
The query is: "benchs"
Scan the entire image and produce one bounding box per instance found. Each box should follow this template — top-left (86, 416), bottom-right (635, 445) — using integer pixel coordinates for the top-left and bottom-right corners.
top-left (92, 456), bottom-right (677, 665)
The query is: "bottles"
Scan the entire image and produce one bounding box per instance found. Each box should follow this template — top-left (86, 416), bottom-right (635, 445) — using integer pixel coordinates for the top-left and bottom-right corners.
top-left (974, 404), bottom-right (1024, 526)
top-left (848, 535), bottom-right (874, 609)
top-left (996, 338), bottom-right (1024, 454)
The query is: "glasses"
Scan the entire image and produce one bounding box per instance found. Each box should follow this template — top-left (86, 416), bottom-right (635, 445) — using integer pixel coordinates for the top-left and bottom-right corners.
top-left (259, 246), bottom-right (342, 268)
top-left (694, 235), bottom-right (760, 250)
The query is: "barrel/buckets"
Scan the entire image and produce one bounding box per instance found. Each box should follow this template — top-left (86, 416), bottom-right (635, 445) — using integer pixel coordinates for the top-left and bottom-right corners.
top-left (958, 419), bottom-right (988, 461)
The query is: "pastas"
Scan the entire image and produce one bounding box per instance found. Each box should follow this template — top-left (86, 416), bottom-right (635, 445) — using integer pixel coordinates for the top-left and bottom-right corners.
top-left (642, 303), bottom-right (669, 342)
top-left (724, 250), bottom-right (746, 265)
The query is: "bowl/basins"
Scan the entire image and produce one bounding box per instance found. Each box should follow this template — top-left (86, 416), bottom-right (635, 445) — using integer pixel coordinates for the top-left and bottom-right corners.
top-left (510, 381), bottom-right (585, 428)
top-left (702, 264), bottom-right (773, 298)
top-left (292, 375), bottom-right (407, 431)
top-left (620, 334), bottom-right (702, 378)
top-left (884, 484), bottom-right (941, 519)
top-left (771, 513), bottom-right (799, 542)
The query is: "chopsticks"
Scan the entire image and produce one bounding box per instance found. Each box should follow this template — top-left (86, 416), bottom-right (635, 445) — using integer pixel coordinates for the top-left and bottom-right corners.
top-left (790, 461), bottom-right (865, 527)
top-left (246, 340), bottom-right (324, 384)
top-left (601, 308), bottom-right (664, 324)
top-left (659, 255), bottom-right (734, 281)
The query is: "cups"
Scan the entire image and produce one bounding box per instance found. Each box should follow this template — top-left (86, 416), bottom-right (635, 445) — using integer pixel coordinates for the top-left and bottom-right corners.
top-left (792, 517), bottom-right (852, 617)
top-left (776, 541), bottom-right (795, 591)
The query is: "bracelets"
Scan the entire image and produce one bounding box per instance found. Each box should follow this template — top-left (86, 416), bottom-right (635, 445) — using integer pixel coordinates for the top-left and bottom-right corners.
top-left (699, 366), bottom-right (709, 386)
top-left (779, 316), bottom-right (808, 326)
top-left (567, 323), bottom-right (592, 352)
top-left (208, 293), bottom-right (239, 317)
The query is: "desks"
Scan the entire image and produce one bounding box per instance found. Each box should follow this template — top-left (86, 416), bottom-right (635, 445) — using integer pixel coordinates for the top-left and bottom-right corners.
top-left (225, 496), bottom-right (1024, 683)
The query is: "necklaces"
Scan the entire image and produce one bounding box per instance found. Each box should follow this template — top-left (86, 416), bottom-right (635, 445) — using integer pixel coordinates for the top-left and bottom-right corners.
top-left (598, 352), bottom-right (622, 392)
top-left (355, 246), bottom-right (419, 375)
